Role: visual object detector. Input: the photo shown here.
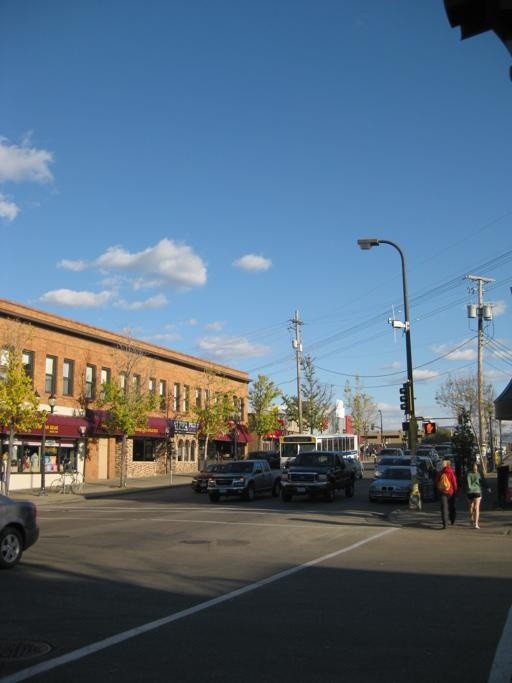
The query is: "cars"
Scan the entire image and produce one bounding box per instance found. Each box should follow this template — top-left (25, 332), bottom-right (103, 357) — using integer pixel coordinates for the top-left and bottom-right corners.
top-left (191, 463), bottom-right (223, 491)
top-left (348, 459), bottom-right (365, 478)
top-left (0, 491), bottom-right (40, 569)
top-left (367, 437), bottom-right (507, 505)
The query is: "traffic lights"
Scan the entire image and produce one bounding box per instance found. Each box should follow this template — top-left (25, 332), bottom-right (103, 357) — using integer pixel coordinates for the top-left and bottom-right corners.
top-left (423, 421), bottom-right (438, 436)
top-left (400, 383), bottom-right (410, 412)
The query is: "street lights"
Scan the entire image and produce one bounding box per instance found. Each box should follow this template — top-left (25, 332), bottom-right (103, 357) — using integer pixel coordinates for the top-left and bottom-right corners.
top-left (32, 390), bottom-right (60, 498)
top-left (375, 408), bottom-right (384, 435)
top-left (356, 233), bottom-right (421, 455)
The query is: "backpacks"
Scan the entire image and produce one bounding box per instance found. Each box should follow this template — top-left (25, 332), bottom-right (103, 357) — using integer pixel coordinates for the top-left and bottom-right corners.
top-left (438, 473), bottom-right (450, 491)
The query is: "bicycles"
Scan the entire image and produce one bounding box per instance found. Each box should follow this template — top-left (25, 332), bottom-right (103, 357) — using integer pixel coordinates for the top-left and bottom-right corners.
top-left (50, 471), bottom-right (87, 498)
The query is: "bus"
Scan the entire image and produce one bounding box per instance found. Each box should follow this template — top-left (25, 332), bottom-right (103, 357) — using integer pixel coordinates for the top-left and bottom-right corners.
top-left (280, 433), bottom-right (359, 482)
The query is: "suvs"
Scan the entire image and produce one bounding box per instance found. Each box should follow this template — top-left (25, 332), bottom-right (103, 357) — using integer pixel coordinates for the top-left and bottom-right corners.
top-left (244, 450), bottom-right (279, 469)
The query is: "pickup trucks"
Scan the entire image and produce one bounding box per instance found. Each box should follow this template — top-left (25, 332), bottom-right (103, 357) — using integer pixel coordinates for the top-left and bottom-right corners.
top-left (208, 459), bottom-right (280, 500)
top-left (282, 450), bottom-right (361, 500)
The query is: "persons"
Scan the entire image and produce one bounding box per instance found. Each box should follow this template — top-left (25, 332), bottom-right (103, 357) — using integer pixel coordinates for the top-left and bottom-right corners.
top-left (464, 464), bottom-right (482, 529)
top-left (435, 460), bottom-right (458, 528)
